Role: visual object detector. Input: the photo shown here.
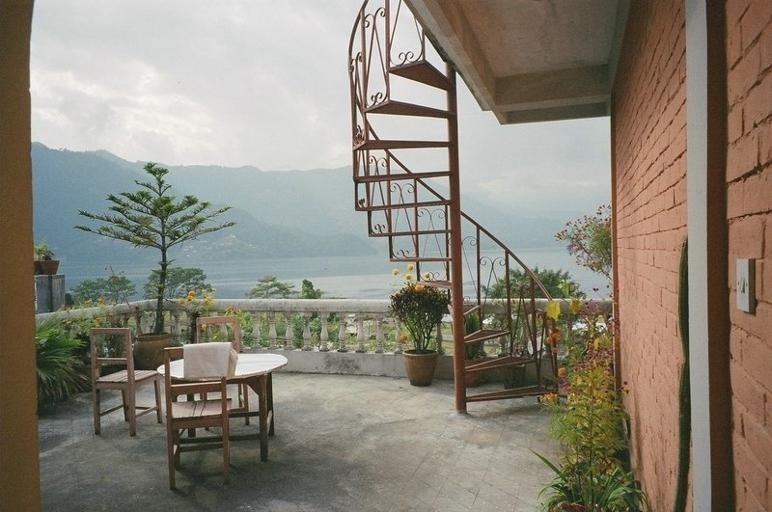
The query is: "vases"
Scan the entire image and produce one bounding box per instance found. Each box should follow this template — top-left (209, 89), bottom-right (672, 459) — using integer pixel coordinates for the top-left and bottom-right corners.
top-left (402, 349), bottom-right (439, 386)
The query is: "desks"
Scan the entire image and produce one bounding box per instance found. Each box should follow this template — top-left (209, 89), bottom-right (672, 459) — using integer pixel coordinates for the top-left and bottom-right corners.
top-left (157, 354), bottom-right (288, 461)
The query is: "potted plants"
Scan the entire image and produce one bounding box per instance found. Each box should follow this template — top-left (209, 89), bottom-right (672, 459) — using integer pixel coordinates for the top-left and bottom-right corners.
top-left (532, 450), bottom-right (646, 512)
top-left (35, 242), bottom-right (60, 275)
top-left (464, 313), bottom-right (486, 387)
top-left (496, 298), bottom-right (526, 388)
top-left (70, 163), bottom-right (234, 370)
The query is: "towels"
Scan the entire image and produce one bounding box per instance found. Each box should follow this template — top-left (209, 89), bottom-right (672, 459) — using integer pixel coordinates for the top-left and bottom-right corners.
top-left (184, 341), bottom-right (238, 378)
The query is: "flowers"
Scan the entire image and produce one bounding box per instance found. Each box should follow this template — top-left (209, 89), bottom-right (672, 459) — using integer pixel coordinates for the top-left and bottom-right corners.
top-left (388, 264), bottom-right (450, 352)
top-left (174, 288), bottom-right (214, 312)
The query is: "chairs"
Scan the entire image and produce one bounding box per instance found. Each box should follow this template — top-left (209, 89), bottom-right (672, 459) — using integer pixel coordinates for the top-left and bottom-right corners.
top-left (89, 316), bottom-right (249, 490)
